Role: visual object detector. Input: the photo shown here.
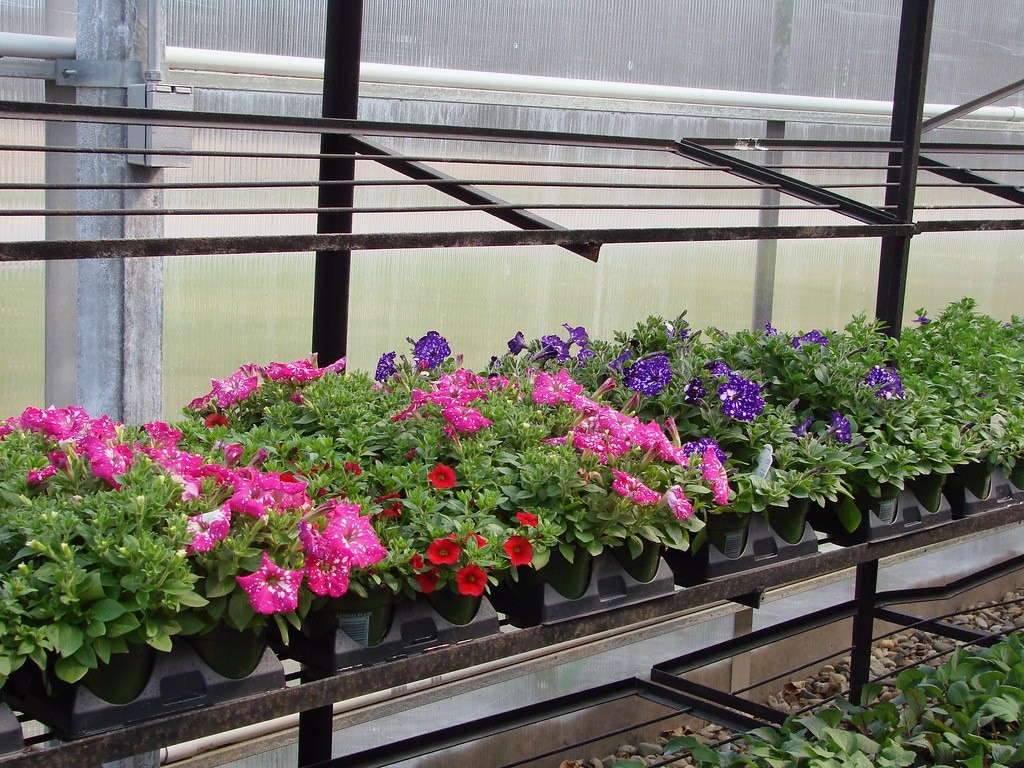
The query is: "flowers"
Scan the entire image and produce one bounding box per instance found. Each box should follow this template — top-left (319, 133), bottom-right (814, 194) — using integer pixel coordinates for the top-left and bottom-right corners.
top-left (0, 298), bottom-right (1024, 589)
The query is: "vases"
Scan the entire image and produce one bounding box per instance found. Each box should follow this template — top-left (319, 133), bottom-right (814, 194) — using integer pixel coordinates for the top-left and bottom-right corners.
top-left (0, 452), bottom-right (1024, 704)
